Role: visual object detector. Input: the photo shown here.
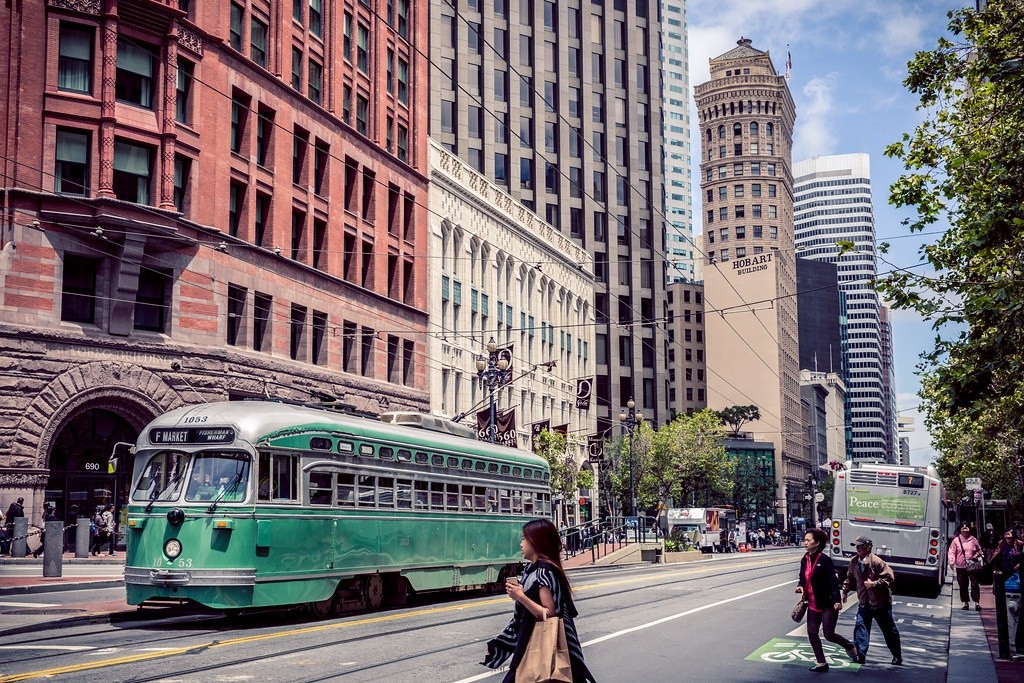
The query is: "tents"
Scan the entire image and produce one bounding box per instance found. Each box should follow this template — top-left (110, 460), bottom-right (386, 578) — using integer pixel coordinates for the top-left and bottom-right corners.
top-left (817, 517), bottom-right (832, 529)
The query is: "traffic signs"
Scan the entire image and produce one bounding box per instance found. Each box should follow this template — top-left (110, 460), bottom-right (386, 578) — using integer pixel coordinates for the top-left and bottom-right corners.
top-left (804, 494), bottom-right (814, 502)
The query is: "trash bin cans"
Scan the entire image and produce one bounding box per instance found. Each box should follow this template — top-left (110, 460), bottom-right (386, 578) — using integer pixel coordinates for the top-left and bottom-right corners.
top-left (567, 529), bottom-right (580, 551)
top-left (582, 526), bottom-right (595, 548)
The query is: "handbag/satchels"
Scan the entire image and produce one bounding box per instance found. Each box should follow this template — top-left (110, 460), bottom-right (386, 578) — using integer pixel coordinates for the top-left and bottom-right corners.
top-left (965, 558), bottom-right (983, 573)
top-left (792, 595), bottom-right (810, 622)
top-left (1003, 570), bottom-right (1021, 592)
top-left (515, 606), bottom-right (573, 683)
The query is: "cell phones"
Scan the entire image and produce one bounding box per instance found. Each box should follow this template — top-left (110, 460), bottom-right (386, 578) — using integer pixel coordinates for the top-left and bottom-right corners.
top-left (506, 577), bottom-right (518, 601)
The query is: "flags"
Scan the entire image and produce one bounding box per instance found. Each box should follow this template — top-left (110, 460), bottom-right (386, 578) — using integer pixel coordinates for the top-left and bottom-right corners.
top-left (786, 51), bottom-right (792, 78)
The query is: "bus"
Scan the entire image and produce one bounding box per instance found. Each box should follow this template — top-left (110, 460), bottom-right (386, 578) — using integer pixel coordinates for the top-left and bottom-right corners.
top-left (828, 462), bottom-right (951, 598)
top-left (108, 400), bottom-right (553, 613)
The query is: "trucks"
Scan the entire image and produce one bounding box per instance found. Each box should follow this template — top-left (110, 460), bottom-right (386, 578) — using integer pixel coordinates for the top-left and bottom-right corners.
top-left (669, 506), bottom-right (747, 553)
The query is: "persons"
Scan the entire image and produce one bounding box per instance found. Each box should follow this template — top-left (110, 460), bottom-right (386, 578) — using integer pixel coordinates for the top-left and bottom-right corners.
top-left (92, 503), bottom-right (117, 556)
top-left (948, 520), bottom-right (1000, 611)
top-left (4, 497), bottom-right (31, 556)
top-left (988, 515), bottom-right (1024, 595)
top-left (728, 527), bottom-right (741, 552)
top-left (67, 505), bottom-right (81, 544)
top-left (31, 501), bottom-right (58, 558)
top-left (258, 457), bottom-right (277, 499)
top-left (479, 518), bottom-right (597, 683)
top-left (607, 532), bottom-right (618, 543)
top-left (580, 524), bottom-right (587, 549)
top-left (768, 527), bottom-right (780, 545)
top-left (559, 521), bottom-right (567, 551)
top-left (0, 510), bottom-right (10, 556)
top-left (89, 505), bottom-right (105, 556)
top-left (841, 535), bottom-right (902, 664)
top-left (795, 529), bottom-right (860, 672)
top-left (720, 526), bottom-right (727, 551)
top-left (746, 528), bottom-right (766, 548)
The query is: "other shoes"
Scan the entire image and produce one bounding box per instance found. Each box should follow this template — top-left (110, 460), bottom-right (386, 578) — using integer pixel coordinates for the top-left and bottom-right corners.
top-left (976, 605), bottom-right (982, 611)
top-left (891, 655), bottom-right (902, 665)
top-left (962, 606), bottom-right (969, 610)
top-left (1013, 653), bottom-right (1024, 661)
top-left (808, 662), bottom-right (829, 672)
top-left (851, 641), bottom-right (865, 664)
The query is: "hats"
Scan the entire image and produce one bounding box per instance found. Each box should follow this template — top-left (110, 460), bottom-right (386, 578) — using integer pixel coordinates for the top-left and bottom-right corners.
top-left (850, 536), bottom-right (872, 548)
top-left (985, 523), bottom-right (993, 529)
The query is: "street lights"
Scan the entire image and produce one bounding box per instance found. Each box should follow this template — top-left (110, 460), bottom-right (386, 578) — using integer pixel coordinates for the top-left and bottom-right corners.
top-left (756, 456), bottom-right (771, 540)
top-left (475, 335), bottom-right (508, 443)
top-left (618, 395), bottom-right (643, 516)
